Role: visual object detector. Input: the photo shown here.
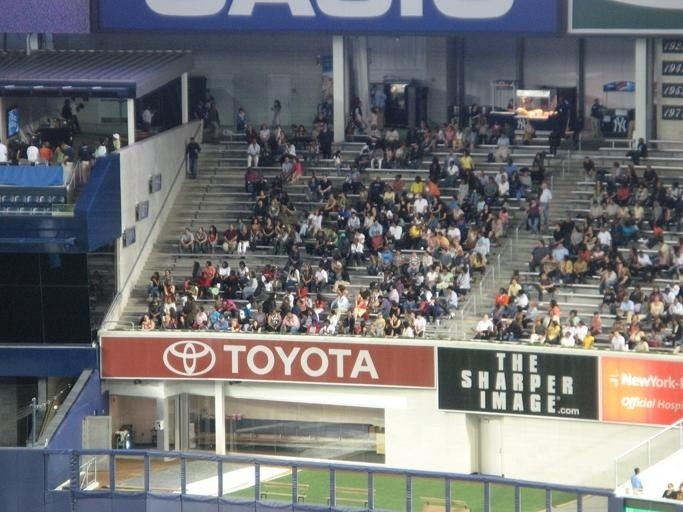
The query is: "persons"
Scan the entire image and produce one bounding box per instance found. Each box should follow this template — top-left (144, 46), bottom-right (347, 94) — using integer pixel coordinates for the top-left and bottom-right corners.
top-left (630, 467), bottom-right (644, 494)
top-left (662, 482), bottom-right (683, 501)
top-left (1, 95), bottom-right (127, 164)
top-left (142, 86), bottom-right (683, 355)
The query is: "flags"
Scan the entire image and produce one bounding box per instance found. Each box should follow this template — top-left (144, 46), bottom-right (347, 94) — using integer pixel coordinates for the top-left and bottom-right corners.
top-left (603, 80), bottom-right (636, 92)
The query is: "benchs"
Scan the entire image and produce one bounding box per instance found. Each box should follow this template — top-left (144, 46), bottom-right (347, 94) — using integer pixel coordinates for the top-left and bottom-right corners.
top-left (118, 130), bottom-right (683, 357)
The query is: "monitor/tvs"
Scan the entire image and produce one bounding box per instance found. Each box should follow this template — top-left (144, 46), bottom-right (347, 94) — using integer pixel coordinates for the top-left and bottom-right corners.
top-left (123, 226), bottom-right (136, 248)
top-left (135, 200), bottom-right (149, 221)
top-left (5, 104), bottom-right (21, 138)
top-left (150, 173), bottom-right (162, 193)
